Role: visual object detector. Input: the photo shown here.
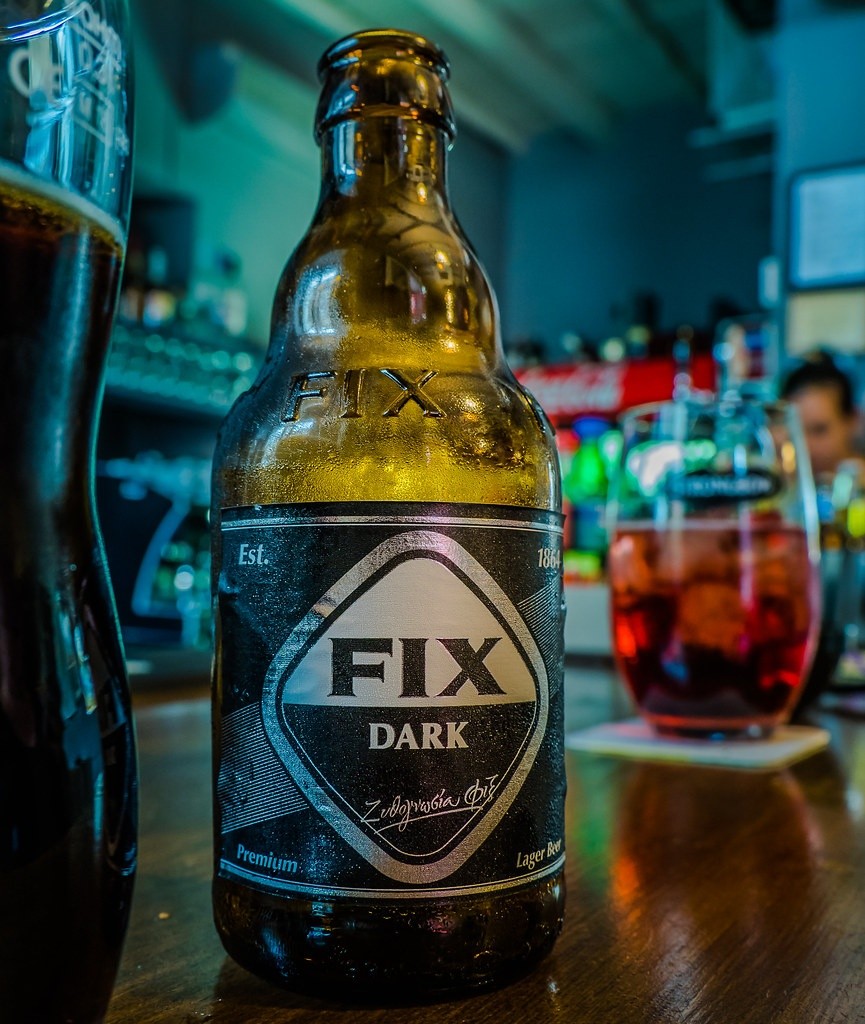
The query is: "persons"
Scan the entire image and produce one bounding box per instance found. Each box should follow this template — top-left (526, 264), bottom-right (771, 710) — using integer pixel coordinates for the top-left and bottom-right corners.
top-left (768, 363), bottom-right (865, 500)
top-left (499, 291), bottom-right (755, 373)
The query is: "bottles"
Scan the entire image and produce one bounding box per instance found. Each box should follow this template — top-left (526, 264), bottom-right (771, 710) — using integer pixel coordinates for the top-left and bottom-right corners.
top-left (214, 26), bottom-right (568, 993)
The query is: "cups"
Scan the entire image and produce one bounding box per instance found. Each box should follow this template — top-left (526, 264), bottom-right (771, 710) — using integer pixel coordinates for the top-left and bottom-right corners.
top-left (0, 0), bottom-right (137, 1024)
top-left (611, 395), bottom-right (821, 739)
